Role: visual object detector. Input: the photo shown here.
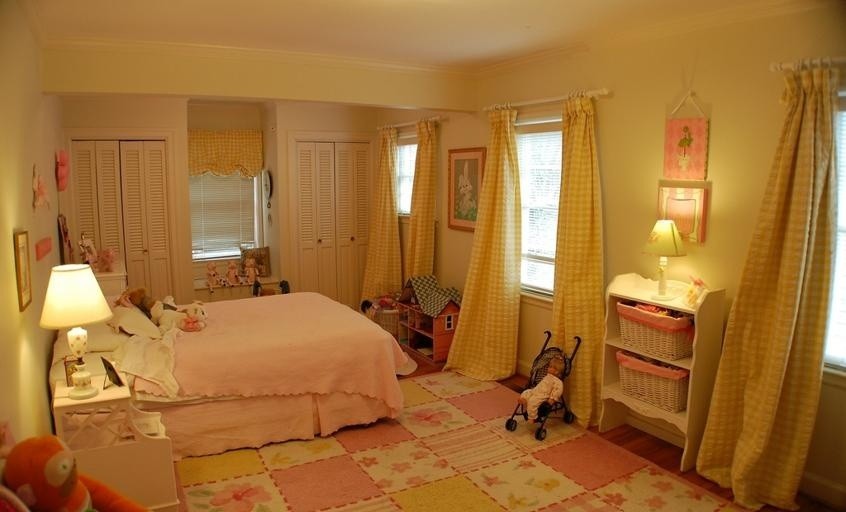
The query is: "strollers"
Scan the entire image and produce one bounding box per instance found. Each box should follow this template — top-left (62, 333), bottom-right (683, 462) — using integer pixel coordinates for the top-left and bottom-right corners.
top-left (504, 330), bottom-right (583, 442)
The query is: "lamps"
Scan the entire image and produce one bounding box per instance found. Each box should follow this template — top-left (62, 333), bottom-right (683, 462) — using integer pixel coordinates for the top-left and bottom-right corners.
top-left (39, 263), bottom-right (113, 399)
top-left (640, 219), bottom-right (686, 301)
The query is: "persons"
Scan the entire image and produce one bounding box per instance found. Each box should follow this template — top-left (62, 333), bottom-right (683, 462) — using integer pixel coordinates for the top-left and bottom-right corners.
top-left (518, 358), bottom-right (564, 424)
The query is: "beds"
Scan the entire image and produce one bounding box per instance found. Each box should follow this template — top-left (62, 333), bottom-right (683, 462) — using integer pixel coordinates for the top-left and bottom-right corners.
top-left (48, 292), bottom-right (417, 463)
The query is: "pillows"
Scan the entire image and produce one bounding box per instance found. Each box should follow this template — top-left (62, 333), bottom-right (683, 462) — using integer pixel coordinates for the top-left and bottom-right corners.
top-left (107, 305), bottom-right (160, 339)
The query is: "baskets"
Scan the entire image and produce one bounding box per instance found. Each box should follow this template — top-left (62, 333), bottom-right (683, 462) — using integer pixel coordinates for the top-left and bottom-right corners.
top-left (616, 299), bottom-right (695, 360)
top-left (374, 308), bottom-right (399, 339)
top-left (616, 349), bottom-right (690, 413)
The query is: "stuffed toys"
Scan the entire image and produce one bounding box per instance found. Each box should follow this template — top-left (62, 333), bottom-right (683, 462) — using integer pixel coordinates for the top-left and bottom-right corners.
top-left (207, 261), bottom-right (225, 294)
top-left (116, 289), bottom-right (161, 324)
top-left (3, 437), bottom-right (150, 512)
top-left (226, 261), bottom-right (243, 287)
top-left (180, 301), bottom-right (208, 332)
top-left (99, 247), bottom-right (115, 272)
top-left (245, 258), bottom-right (261, 286)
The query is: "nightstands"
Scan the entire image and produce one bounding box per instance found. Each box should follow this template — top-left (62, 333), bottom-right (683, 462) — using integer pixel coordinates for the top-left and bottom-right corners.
top-left (93, 260), bottom-right (127, 298)
top-left (53, 373), bottom-right (179, 510)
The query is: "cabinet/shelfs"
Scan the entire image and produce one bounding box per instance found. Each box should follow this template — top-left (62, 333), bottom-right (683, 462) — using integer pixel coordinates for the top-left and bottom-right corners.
top-left (296, 140), bottom-right (369, 313)
top-left (70, 140), bottom-right (121, 261)
top-left (603, 294), bottom-right (695, 434)
top-left (120, 139), bottom-right (173, 301)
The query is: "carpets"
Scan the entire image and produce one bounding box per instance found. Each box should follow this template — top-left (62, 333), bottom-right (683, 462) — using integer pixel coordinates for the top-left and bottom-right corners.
top-left (178, 372), bottom-right (750, 511)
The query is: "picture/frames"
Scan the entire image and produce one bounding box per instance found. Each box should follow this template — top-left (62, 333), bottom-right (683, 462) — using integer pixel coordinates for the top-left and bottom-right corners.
top-left (657, 178), bottom-right (710, 245)
top-left (13, 231), bottom-right (32, 312)
top-left (447, 147), bottom-right (487, 232)
top-left (101, 357), bottom-right (123, 389)
